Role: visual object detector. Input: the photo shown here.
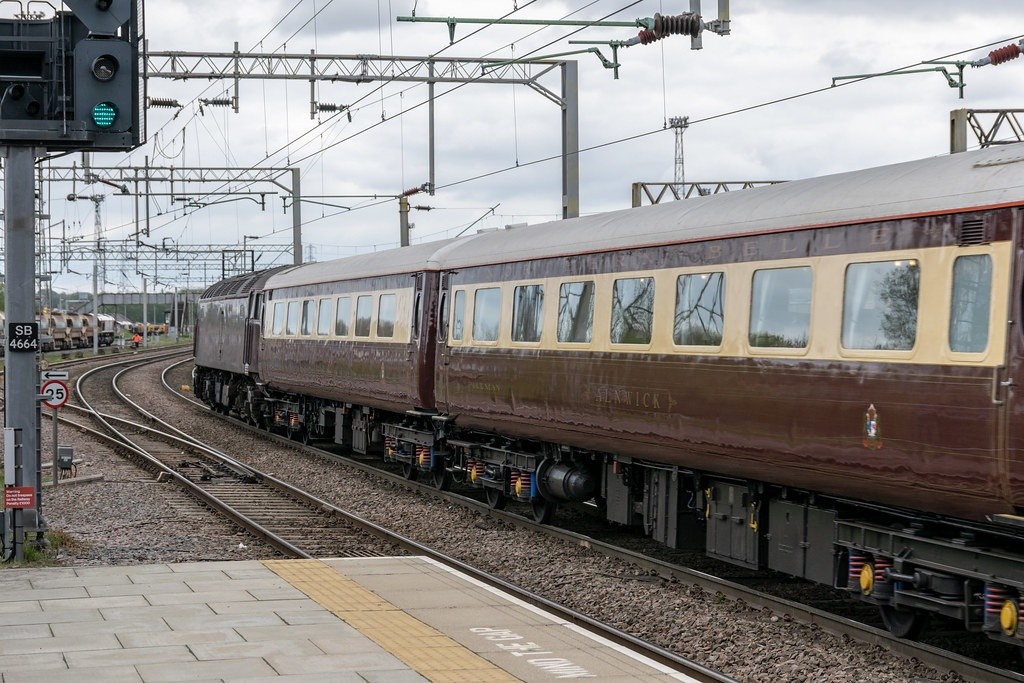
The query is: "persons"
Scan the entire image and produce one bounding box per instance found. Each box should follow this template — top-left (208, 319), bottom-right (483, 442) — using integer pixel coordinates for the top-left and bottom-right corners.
top-left (131, 333), bottom-right (142, 348)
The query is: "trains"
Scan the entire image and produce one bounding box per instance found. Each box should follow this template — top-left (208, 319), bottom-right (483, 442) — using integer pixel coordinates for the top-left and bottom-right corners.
top-left (0, 311), bottom-right (115, 351)
top-left (193, 142), bottom-right (1024, 647)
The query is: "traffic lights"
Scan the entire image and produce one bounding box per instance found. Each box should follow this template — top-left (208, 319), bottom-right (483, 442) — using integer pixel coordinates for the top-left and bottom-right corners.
top-left (62, 0), bottom-right (137, 150)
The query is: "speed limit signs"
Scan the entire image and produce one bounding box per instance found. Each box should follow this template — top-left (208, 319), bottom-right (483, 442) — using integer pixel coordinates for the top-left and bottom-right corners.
top-left (41, 381), bottom-right (68, 408)
